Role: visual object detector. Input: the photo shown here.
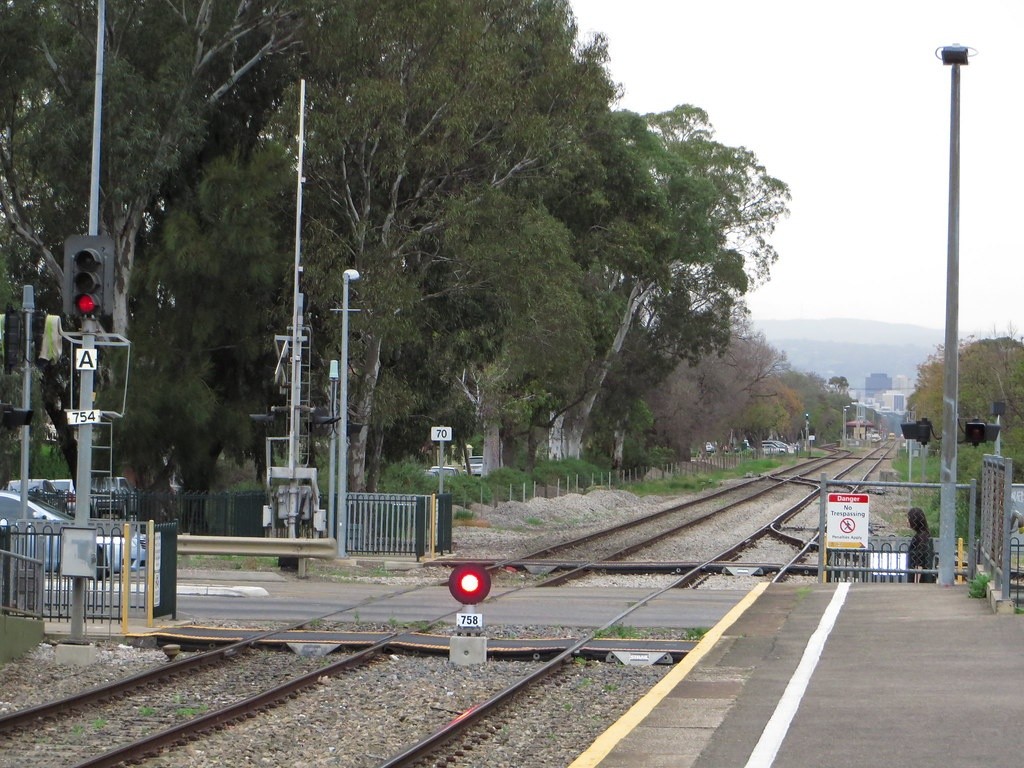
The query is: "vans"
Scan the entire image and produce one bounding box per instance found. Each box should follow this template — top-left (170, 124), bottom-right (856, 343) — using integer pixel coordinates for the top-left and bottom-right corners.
top-left (465, 456), bottom-right (483, 477)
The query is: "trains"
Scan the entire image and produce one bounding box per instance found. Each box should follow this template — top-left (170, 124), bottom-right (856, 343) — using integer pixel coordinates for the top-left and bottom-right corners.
top-left (888, 433), bottom-right (895, 440)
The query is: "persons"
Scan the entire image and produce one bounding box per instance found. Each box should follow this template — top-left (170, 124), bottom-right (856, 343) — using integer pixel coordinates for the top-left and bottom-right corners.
top-left (906, 507), bottom-right (934, 583)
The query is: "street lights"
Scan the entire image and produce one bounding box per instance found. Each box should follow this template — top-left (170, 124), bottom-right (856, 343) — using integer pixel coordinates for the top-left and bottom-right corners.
top-left (329, 269), bottom-right (364, 555)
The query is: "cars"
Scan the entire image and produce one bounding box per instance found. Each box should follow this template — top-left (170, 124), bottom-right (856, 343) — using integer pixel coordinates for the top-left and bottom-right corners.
top-left (6, 476), bottom-right (137, 518)
top-left (762, 440), bottom-right (789, 454)
top-left (423, 466), bottom-right (460, 477)
top-left (0, 489), bottom-right (149, 581)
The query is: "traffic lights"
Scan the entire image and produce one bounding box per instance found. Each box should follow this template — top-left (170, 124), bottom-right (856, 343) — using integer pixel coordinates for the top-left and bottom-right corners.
top-left (0, 302), bottom-right (22, 374)
top-left (32, 309), bottom-right (50, 367)
top-left (448, 565), bottom-right (491, 606)
top-left (61, 235), bottom-right (116, 316)
top-left (964, 418), bottom-right (999, 446)
top-left (0, 402), bottom-right (34, 429)
top-left (806, 413), bottom-right (809, 419)
